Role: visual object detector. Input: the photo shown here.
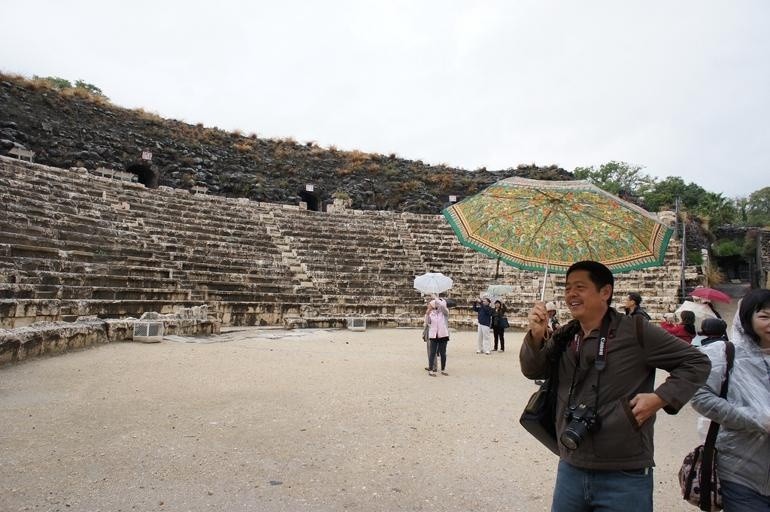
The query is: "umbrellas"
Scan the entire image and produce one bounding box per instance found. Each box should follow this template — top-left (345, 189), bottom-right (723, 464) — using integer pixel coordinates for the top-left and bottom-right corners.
top-left (487, 284), bottom-right (512, 296)
top-left (687, 287), bottom-right (731, 304)
top-left (413, 272), bottom-right (454, 295)
top-left (441, 176), bottom-right (675, 302)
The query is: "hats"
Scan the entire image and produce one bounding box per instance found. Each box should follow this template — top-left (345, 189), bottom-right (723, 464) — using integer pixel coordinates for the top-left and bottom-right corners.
top-left (429, 300), bottom-right (436, 308)
top-left (703, 317), bottom-right (727, 336)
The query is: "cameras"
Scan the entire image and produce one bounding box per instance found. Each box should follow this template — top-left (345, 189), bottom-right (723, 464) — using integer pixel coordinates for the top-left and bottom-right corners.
top-left (560, 403), bottom-right (602, 450)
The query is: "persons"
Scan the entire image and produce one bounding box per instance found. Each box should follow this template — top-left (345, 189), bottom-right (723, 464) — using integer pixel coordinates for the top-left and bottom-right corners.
top-left (425, 294), bottom-right (449, 377)
top-left (625, 292), bottom-right (651, 320)
top-left (472, 298), bottom-right (493, 355)
top-left (424, 304), bottom-right (438, 372)
top-left (544, 302), bottom-right (561, 331)
top-left (519, 261), bottom-right (711, 512)
top-left (690, 288), bottom-right (769, 511)
top-left (660, 301), bottom-right (728, 345)
top-left (490, 300), bottom-right (507, 352)
top-left (437, 344), bottom-right (441, 356)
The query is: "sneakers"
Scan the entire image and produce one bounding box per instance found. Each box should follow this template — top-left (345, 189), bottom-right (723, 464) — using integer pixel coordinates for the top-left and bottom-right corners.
top-left (477, 348), bottom-right (503, 354)
top-left (425, 366), bottom-right (449, 377)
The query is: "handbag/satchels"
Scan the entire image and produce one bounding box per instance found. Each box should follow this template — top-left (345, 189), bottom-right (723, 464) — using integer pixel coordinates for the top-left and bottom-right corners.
top-left (678, 445), bottom-right (722, 512)
top-left (498, 317), bottom-right (510, 328)
top-left (422, 324), bottom-right (430, 342)
top-left (519, 378), bottom-right (561, 456)
